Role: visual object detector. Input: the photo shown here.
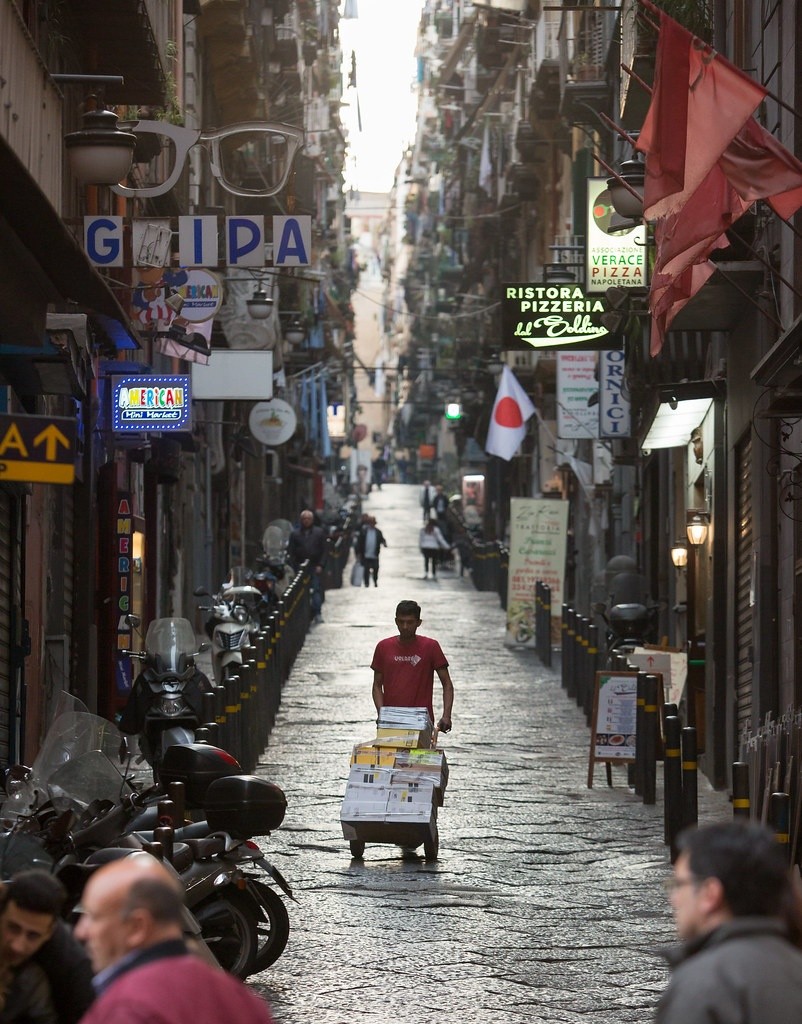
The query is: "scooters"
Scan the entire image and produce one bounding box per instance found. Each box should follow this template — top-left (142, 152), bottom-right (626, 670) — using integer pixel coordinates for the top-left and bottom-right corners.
top-left (589, 555), bottom-right (664, 670)
top-left (197, 518), bottom-right (297, 684)
top-left (120, 612), bottom-right (215, 785)
top-left (0, 685), bottom-right (300, 984)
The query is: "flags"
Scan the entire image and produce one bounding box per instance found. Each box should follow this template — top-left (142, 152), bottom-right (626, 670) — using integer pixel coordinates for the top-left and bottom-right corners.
top-left (484, 363), bottom-right (535, 461)
top-left (636, 11), bottom-right (802, 357)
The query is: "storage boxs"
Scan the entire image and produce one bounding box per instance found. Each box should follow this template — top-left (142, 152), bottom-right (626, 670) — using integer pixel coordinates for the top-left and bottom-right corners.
top-left (159, 744), bottom-right (244, 809)
top-left (608, 603), bottom-right (649, 635)
top-left (340, 706), bottom-right (449, 822)
top-left (205, 776), bottom-right (287, 836)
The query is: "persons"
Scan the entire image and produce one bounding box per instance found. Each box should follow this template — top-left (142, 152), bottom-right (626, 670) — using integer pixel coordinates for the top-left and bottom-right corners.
top-left (418, 480), bottom-right (436, 523)
top-left (419, 519), bottom-right (451, 580)
top-left (0, 871), bottom-right (98, 1024)
top-left (74, 852), bottom-right (275, 1024)
top-left (651, 818), bottom-right (802, 1024)
top-left (434, 485), bottom-right (450, 521)
top-left (287, 509), bottom-right (325, 622)
top-left (370, 600), bottom-right (455, 734)
top-left (350, 503), bottom-right (388, 586)
top-left (373, 454), bottom-right (386, 490)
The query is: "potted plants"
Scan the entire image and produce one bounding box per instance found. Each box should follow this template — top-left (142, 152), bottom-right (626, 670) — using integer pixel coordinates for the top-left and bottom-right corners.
top-left (117, 39), bottom-right (184, 162)
top-left (577, 52), bottom-right (601, 80)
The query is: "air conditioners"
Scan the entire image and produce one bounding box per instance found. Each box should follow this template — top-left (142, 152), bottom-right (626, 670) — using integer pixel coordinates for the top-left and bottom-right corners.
top-left (266, 450), bottom-right (280, 478)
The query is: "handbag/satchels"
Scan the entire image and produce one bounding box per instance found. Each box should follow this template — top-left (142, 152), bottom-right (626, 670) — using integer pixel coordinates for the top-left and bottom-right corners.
top-left (350, 562), bottom-right (365, 585)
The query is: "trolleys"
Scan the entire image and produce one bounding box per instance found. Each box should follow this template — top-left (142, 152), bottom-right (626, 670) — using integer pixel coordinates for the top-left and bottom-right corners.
top-left (349, 724), bottom-right (439, 861)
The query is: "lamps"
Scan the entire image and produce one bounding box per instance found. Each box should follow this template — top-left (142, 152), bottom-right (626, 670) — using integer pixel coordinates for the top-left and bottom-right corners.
top-left (605, 285), bottom-right (648, 309)
top-left (545, 246), bottom-right (586, 282)
top-left (687, 512), bottom-right (711, 548)
top-left (278, 311), bottom-right (304, 344)
top-left (600, 310), bottom-right (650, 333)
top-left (225, 277), bottom-right (274, 318)
top-left (670, 537), bottom-right (688, 570)
top-left (50, 74), bottom-right (138, 186)
top-left (487, 353), bottom-right (503, 376)
top-left (607, 150), bottom-right (645, 222)
top-left (195, 420), bottom-right (259, 457)
top-left (110, 282), bottom-right (185, 314)
top-left (137, 325), bottom-right (211, 356)
top-left (461, 386), bottom-right (477, 401)
